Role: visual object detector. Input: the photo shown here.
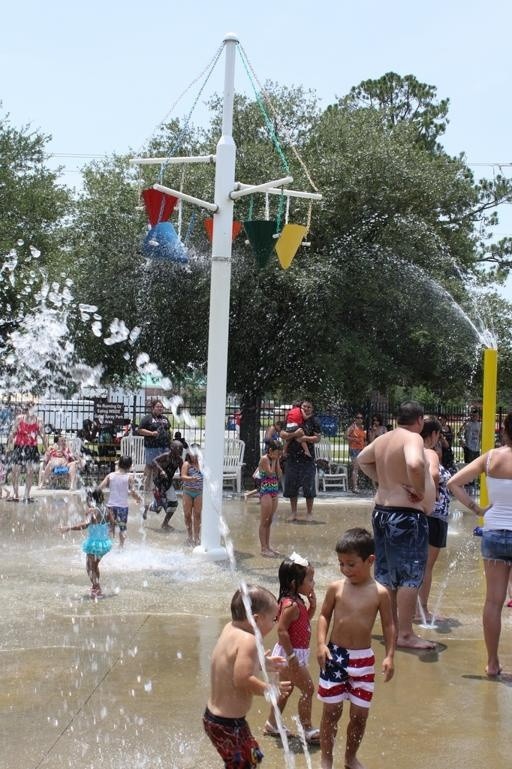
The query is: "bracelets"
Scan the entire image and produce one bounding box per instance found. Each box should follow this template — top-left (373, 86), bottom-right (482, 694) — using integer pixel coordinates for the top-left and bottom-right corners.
top-left (476, 508), bottom-right (482, 515)
top-left (467, 501), bottom-right (475, 508)
top-left (284, 652), bottom-right (296, 662)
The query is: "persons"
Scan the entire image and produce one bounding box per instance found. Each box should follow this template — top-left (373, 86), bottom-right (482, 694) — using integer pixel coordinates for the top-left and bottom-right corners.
top-left (201, 585), bottom-right (292, 768)
top-left (315, 525), bottom-right (399, 769)
top-left (263, 555), bottom-right (324, 743)
top-left (444, 413), bottom-right (510, 678)
top-left (415, 413), bottom-right (450, 623)
top-left (356, 399), bottom-right (442, 649)
top-left (233, 395), bottom-right (323, 523)
top-left (343, 398), bottom-right (483, 495)
top-left (257, 437), bottom-right (284, 560)
top-left (2, 396), bottom-right (203, 549)
top-left (57, 487), bottom-right (119, 600)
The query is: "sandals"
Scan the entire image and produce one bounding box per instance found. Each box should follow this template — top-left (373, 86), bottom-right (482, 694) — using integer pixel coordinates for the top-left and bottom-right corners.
top-left (297, 728), bottom-right (321, 746)
top-left (262, 719), bottom-right (296, 738)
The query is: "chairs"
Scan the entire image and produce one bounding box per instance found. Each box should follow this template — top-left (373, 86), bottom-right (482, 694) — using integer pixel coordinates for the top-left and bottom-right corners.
top-left (222, 438), bottom-right (246, 493)
top-left (313, 437), bottom-right (350, 491)
top-left (453, 461), bottom-right (479, 496)
top-left (114, 435), bottom-right (146, 496)
top-left (38, 436), bottom-right (82, 489)
